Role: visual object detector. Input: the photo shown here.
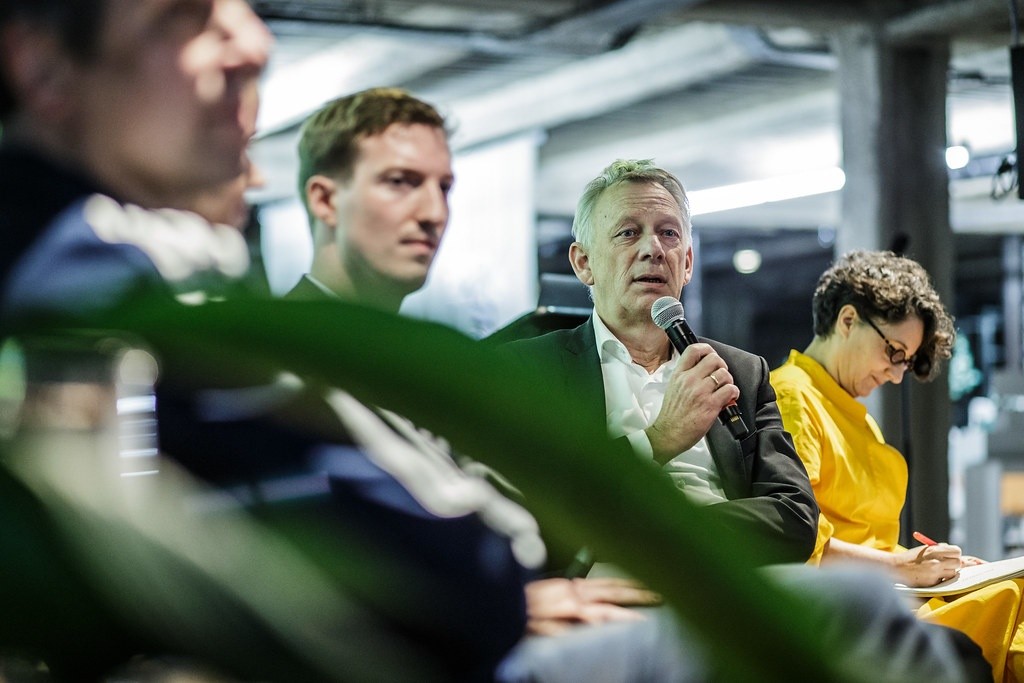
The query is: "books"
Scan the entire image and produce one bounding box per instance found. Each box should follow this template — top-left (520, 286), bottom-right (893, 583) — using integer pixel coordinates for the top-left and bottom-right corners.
top-left (894, 553), bottom-right (1023, 597)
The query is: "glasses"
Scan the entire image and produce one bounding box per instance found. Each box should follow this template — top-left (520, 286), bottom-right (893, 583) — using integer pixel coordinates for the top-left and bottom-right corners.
top-left (857, 308), bottom-right (915, 374)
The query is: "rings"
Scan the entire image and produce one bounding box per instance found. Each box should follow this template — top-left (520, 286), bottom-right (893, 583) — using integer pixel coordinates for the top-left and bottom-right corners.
top-left (709, 374), bottom-right (719, 385)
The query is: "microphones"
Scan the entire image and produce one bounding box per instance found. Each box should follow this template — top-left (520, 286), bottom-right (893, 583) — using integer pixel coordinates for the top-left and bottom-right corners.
top-left (651, 295), bottom-right (749, 440)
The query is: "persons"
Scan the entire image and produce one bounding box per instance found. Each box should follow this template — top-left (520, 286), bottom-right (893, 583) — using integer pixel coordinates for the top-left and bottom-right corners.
top-left (495, 152), bottom-right (993, 683)
top-left (266, 86), bottom-right (562, 579)
top-left (0, 0), bottom-right (969, 683)
top-left (764, 245), bottom-right (1024, 683)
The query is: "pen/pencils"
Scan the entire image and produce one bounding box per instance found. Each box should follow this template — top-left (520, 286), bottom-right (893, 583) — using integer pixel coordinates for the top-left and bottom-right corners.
top-left (912, 531), bottom-right (937, 546)
